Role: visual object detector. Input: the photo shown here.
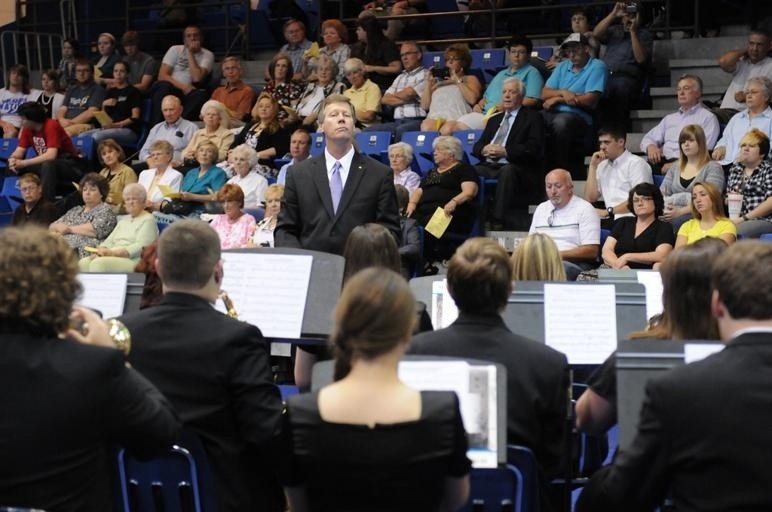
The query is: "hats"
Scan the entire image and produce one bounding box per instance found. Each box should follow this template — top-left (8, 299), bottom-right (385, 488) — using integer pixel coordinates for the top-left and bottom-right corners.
top-left (560, 32), bottom-right (588, 46)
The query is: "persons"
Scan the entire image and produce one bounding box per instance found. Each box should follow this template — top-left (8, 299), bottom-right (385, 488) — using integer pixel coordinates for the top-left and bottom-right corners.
top-left (293, 222), bottom-right (435, 393)
top-left (109, 219), bottom-right (284, 510)
top-left (640, 1), bottom-right (772, 248)
top-left (0, 0), bottom-right (772, 282)
top-left (599, 238), bottom-right (771, 509)
top-left (575, 242), bottom-right (728, 510)
top-left (0, 224), bottom-right (181, 512)
top-left (598, 182), bottom-right (675, 271)
top-left (399, 238), bottom-right (570, 510)
top-left (264, 268), bottom-right (469, 510)
top-left (1, 1), bottom-right (654, 273)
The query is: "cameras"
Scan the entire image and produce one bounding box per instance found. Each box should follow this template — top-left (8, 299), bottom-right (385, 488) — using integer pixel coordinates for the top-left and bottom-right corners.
top-left (430, 68), bottom-right (451, 78)
top-left (625, 1), bottom-right (639, 13)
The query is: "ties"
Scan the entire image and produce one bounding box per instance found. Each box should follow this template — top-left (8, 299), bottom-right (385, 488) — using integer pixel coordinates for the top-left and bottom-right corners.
top-left (547, 208), bottom-right (556, 226)
top-left (330, 162), bottom-right (344, 216)
top-left (492, 112), bottom-right (512, 145)
